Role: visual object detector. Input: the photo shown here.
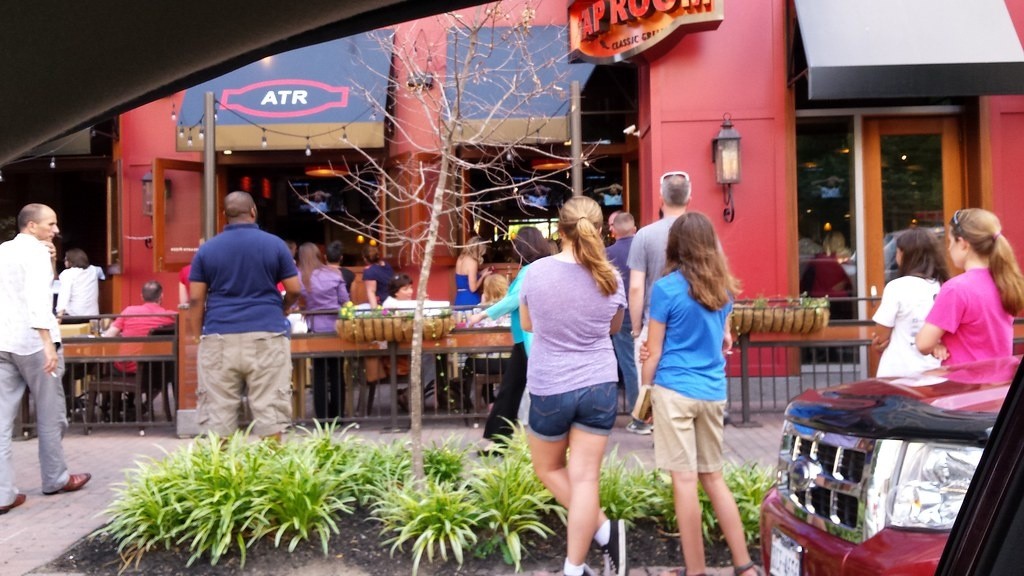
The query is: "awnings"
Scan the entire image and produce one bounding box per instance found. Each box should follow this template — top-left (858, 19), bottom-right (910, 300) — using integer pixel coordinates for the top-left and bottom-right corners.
top-left (176, 29), bottom-right (394, 151)
top-left (786, 0), bottom-right (1024, 100)
top-left (443, 24), bottom-right (638, 144)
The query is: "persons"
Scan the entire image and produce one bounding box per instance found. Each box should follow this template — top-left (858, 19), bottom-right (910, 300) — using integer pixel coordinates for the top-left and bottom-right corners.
top-left (798, 225), bottom-right (852, 320)
top-left (603, 211), bottom-right (654, 435)
top-left (362, 245), bottom-right (394, 308)
top-left (916, 208), bottom-right (1024, 366)
top-left (872, 228), bottom-right (951, 378)
top-left (638, 212), bottom-right (761, 576)
top-left (104, 281), bottom-right (178, 376)
top-left (55, 249), bottom-right (105, 325)
top-left (465, 274), bottom-right (508, 402)
top-left (455, 235), bottom-right (493, 312)
top-left (470, 227), bottom-right (552, 457)
top-left (324, 241), bottom-right (356, 292)
top-left (625, 172), bottom-right (732, 387)
top-left (383, 272), bottom-right (413, 413)
top-left (0, 204), bottom-right (92, 515)
top-left (179, 265), bottom-right (192, 306)
top-left (299, 243), bottom-right (350, 427)
top-left (518, 196), bottom-right (629, 576)
top-left (189, 191), bottom-right (300, 448)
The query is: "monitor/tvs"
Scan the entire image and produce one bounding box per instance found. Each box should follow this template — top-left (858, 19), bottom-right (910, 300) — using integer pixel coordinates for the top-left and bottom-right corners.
top-left (286, 181), bottom-right (350, 214)
top-left (505, 175), bottom-right (624, 211)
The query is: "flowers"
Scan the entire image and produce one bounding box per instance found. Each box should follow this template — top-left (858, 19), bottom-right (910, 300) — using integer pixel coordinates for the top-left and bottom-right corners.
top-left (339, 300), bottom-right (451, 319)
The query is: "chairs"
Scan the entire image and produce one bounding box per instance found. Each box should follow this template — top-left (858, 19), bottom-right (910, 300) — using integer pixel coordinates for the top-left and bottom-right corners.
top-left (293, 358), bottom-right (503, 415)
top-left (83, 325), bottom-right (175, 436)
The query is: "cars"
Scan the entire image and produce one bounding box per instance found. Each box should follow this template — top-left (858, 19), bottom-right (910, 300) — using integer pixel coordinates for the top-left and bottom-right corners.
top-left (759, 355), bottom-right (1024, 576)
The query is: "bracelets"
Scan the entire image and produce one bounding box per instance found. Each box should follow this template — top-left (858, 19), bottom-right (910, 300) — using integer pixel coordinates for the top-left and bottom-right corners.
top-left (631, 331), bottom-right (639, 338)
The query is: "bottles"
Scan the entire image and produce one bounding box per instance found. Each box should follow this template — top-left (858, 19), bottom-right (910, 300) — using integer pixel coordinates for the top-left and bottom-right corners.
top-left (506, 266), bottom-right (512, 284)
top-left (462, 315), bottom-right (466, 324)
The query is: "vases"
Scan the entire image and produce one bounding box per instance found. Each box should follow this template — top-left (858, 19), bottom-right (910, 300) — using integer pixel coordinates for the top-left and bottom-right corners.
top-left (336, 318), bottom-right (454, 342)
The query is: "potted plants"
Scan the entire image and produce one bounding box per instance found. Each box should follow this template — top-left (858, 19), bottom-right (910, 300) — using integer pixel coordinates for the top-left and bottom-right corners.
top-left (730, 296), bottom-right (832, 334)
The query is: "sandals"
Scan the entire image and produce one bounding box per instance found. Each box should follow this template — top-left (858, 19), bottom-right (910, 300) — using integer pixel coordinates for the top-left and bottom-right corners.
top-left (734, 561), bottom-right (762, 576)
top-left (657, 569), bottom-right (706, 576)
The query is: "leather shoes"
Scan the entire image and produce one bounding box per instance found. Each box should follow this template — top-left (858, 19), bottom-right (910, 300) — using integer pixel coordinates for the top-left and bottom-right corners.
top-left (44, 473), bottom-right (91, 495)
top-left (0, 494), bottom-right (26, 514)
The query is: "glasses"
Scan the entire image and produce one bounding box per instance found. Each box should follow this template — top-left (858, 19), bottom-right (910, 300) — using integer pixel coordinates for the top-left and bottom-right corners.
top-left (949, 210), bottom-right (966, 239)
top-left (660, 171), bottom-right (690, 185)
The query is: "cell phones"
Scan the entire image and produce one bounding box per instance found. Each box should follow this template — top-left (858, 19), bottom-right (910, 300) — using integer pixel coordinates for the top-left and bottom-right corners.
top-left (488, 266), bottom-right (495, 272)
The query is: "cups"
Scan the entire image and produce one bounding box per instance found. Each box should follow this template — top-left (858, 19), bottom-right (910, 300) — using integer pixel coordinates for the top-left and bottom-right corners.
top-left (453, 311), bottom-right (462, 324)
top-left (44, 363), bottom-right (64, 378)
top-left (102, 318), bottom-right (111, 331)
top-left (90, 319), bottom-right (99, 336)
top-left (473, 308), bottom-right (481, 317)
top-left (465, 312), bottom-right (472, 322)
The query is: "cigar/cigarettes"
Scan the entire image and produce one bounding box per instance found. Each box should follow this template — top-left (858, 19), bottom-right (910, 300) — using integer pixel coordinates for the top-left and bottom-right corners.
top-left (51, 372), bottom-right (57, 378)
top-left (727, 351), bottom-right (733, 355)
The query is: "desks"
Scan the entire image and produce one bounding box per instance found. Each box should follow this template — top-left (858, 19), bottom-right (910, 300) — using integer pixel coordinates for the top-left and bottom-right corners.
top-left (58, 318), bottom-right (111, 419)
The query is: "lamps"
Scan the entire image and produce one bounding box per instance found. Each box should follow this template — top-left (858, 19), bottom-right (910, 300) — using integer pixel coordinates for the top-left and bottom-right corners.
top-left (711, 112), bottom-right (742, 224)
top-left (531, 160), bottom-right (572, 170)
top-left (139, 170), bottom-right (172, 250)
top-left (304, 166), bottom-right (348, 177)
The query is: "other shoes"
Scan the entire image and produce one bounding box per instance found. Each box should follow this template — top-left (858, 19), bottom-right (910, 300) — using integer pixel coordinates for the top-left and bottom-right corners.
top-left (397, 389), bottom-right (408, 412)
top-left (478, 445), bottom-right (507, 457)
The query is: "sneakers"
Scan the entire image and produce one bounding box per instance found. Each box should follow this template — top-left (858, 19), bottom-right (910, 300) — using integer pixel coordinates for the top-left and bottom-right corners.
top-left (594, 519), bottom-right (625, 576)
top-left (627, 421), bottom-right (655, 435)
